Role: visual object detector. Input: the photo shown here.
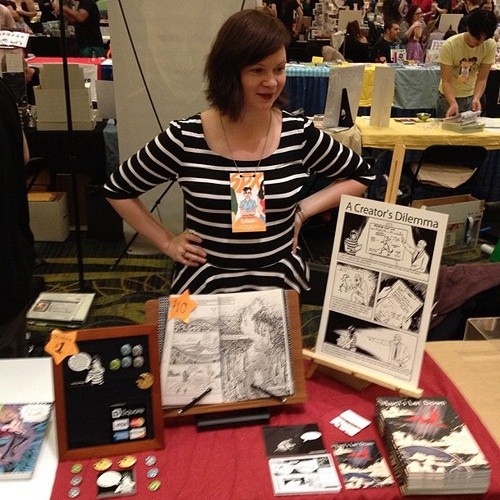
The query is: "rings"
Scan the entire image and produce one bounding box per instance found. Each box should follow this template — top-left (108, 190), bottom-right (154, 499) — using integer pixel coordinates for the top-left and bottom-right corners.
top-left (182, 250), bottom-right (186, 256)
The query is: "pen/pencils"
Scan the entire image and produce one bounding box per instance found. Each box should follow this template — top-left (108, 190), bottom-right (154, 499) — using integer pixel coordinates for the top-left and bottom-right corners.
top-left (178, 388), bottom-right (211, 415)
top-left (252, 383), bottom-right (282, 404)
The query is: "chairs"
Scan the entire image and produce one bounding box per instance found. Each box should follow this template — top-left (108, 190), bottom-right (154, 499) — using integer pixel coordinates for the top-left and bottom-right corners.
top-left (410, 145), bottom-right (488, 200)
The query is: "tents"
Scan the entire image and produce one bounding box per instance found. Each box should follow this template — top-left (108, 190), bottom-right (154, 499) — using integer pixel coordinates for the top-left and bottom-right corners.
top-left (25, 291), bottom-right (96, 323)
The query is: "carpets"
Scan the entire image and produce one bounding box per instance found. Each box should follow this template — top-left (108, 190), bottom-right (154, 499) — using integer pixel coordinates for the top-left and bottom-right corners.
top-left (45, 256), bottom-right (323, 349)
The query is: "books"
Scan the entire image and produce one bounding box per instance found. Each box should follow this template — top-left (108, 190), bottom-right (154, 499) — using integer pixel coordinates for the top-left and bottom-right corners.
top-left (372, 397), bottom-right (491, 495)
top-left (160, 289), bottom-right (294, 405)
top-left (443, 110), bottom-right (486, 134)
top-left (267, 454), bottom-right (341, 496)
top-left (331, 436), bottom-right (396, 490)
top-left (0, 402), bottom-right (54, 480)
top-left (262, 422), bottom-right (325, 458)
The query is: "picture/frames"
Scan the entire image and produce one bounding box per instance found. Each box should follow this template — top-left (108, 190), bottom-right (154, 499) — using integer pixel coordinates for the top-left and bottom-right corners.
top-left (45, 323), bottom-right (166, 462)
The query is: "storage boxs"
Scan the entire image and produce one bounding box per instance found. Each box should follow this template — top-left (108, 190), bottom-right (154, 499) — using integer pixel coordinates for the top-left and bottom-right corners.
top-left (411, 194), bottom-right (485, 256)
top-left (26, 192), bottom-right (70, 243)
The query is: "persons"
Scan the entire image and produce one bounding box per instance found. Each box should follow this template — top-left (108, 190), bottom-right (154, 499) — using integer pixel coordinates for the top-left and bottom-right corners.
top-left (2, 56), bottom-right (41, 105)
top-left (102, 7), bottom-right (380, 307)
top-left (369, 19), bottom-right (404, 64)
top-left (270, 0), bottom-right (304, 62)
top-left (295, 0), bottom-right (500, 43)
top-left (434, 10), bottom-right (498, 232)
top-left (339, 20), bottom-right (371, 63)
top-left (51, 0), bottom-right (104, 58)
top-left (1, 75), bottom-right (51, 348)
top-left (398, 5), bottom-right (430, 63)
top-left (1, 0), bottom-right (62, 36)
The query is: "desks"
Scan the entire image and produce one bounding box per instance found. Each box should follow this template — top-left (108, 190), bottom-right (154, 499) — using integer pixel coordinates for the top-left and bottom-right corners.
top-left (274, 62), bottom-right (333, 118)
top-left (360, 61), bottom-right (443, 117)
top-left (0, 340), bottom-right (500, 500)
top-left (311, 117), bottom-right (500, 204)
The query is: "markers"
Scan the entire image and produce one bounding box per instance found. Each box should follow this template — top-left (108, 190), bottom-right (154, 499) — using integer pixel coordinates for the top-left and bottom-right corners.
top-left (196, 415), bottom-right (270, 432)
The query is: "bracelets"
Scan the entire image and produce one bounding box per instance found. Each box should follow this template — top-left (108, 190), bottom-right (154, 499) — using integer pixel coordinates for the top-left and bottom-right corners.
top-left (294, 202), bottom-right (306, 224)
